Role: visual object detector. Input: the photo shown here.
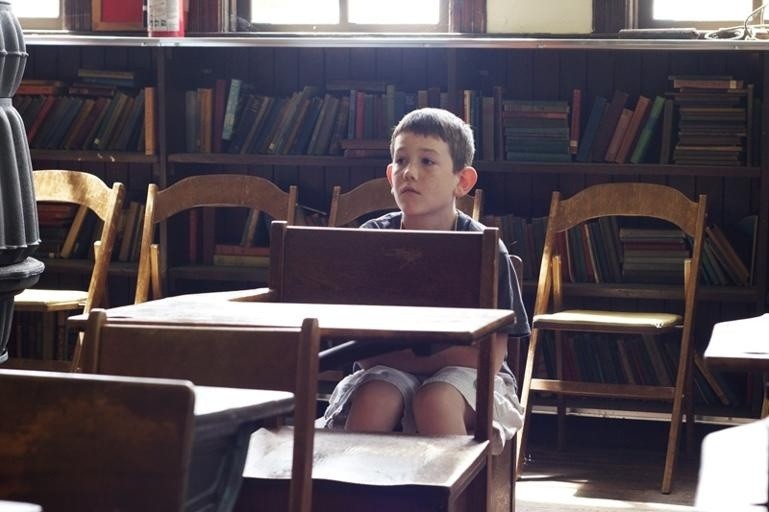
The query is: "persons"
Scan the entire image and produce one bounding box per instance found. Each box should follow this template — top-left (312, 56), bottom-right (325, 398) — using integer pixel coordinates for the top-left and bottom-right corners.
top-left (320, 106), bottom-right (530, 457)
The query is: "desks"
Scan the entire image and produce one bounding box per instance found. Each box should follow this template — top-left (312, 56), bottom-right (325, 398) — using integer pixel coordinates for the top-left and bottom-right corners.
top-left (692, 312), bottom-right (769, 511)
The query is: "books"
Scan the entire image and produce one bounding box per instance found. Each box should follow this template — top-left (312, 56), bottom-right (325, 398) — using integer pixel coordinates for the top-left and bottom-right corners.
top-left (317, 82), bottom-right (448, 160)
top-left (464, 70), bottom-right (759, 163)
top-left (14, 310), bottom-right (80, 362)
top-left (535, 331), bottom-right (760, 407)
top-left (480, 213), bottom-right (760, 284)
top-left (13, 67), bottom-right (326, 269)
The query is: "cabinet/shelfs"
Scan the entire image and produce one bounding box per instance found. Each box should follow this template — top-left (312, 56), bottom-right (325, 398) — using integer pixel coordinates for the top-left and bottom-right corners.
top-left (13, 38), bottom-right (769, 426)
